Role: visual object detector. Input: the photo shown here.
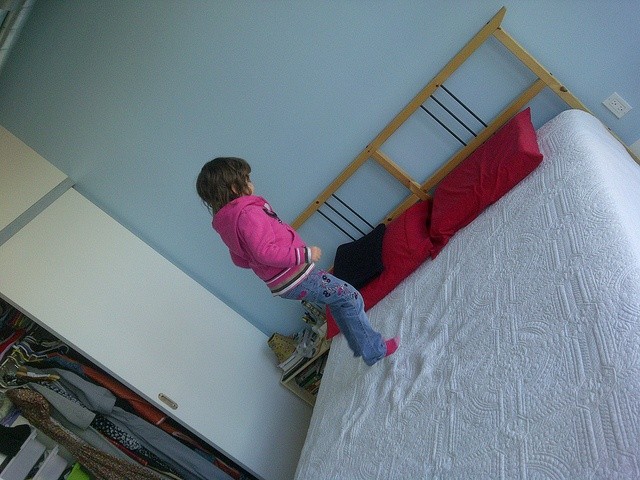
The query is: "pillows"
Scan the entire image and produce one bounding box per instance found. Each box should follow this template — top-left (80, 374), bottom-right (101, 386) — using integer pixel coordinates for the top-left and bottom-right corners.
top-left (429, 107), bottom-right (544, 255)
top-left (325, 200), bottom-right (435, 340)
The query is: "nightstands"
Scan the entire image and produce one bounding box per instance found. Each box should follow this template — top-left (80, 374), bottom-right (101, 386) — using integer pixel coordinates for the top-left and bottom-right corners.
top-left (280, 335), bottom-right (332, 408)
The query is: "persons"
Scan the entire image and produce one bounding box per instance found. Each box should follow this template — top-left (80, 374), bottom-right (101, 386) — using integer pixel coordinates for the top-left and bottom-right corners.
top-left (194, 155), bottom-right (401, 368)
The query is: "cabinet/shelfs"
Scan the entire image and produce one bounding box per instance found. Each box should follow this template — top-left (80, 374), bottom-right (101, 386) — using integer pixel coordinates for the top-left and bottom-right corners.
top-left (0, 123), bottom-right (314, 479)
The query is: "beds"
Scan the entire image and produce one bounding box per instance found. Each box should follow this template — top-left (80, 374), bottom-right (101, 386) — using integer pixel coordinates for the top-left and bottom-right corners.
top-left (291, 7), bottom-right (640, 480)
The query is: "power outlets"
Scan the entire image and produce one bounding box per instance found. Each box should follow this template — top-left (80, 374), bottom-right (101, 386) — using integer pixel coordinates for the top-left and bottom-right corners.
top-left (601, 91), bottom-right (633, 119)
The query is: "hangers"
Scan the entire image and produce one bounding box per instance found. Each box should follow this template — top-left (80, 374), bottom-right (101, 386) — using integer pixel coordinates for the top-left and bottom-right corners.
top-left (0, 330), bottom-right (67, 391)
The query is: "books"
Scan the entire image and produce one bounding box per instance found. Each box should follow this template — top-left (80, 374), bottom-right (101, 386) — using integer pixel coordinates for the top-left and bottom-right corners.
top-left (276, 327), bottom-right (314, 376)
top-left (294, 351), bottom-right (328, 398)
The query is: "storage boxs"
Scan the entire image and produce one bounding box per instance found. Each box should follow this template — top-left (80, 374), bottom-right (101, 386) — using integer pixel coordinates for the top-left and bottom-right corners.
top-left (33, 444), bottom-right (68, 480)
top-left (0, 428), bottom-right (47, 480)
